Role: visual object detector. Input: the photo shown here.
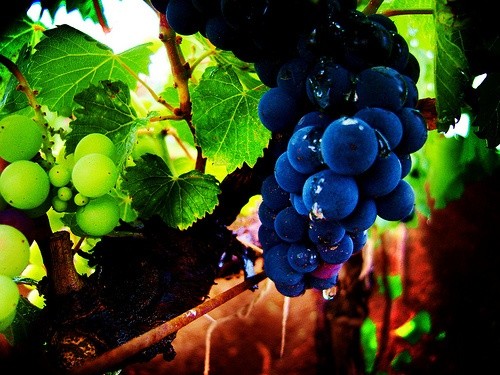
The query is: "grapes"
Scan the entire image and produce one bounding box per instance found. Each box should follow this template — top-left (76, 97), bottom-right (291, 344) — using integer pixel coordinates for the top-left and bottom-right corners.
top-left (0, 115), bottom-right (121, 333)
top-left (151, 1), bottom-right (428, 298)
